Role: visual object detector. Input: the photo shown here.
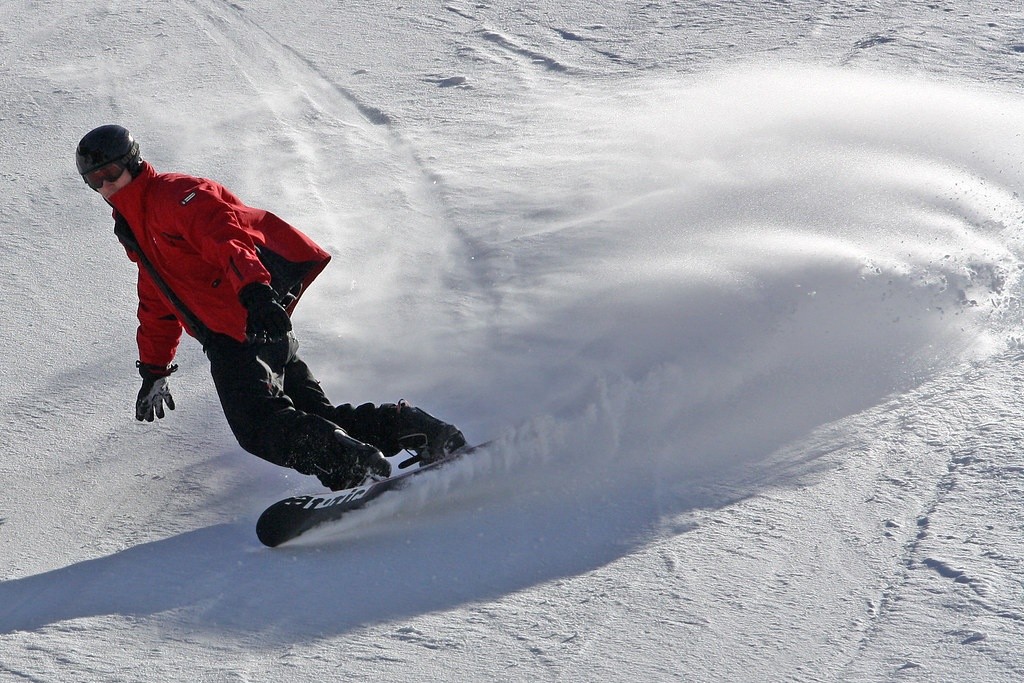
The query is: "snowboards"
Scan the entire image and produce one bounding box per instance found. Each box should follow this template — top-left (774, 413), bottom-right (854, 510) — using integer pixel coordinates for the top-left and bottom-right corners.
top-left (255, 439), bottom-right (495, 549)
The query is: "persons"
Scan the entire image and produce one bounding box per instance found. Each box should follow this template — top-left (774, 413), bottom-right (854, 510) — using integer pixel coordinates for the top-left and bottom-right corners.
top-left (75, 124), bottom-right (467, 491)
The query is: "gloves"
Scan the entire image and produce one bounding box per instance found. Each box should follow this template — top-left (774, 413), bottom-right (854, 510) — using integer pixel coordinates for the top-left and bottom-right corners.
top-left (135, 360), bottom-right (178, 422)
top-left (238, 281), bottom-right (292, 346)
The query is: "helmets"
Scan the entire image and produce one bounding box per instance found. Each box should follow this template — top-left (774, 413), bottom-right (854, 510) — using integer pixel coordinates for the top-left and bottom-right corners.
top-left (75, 124), bottom-right (140, 193)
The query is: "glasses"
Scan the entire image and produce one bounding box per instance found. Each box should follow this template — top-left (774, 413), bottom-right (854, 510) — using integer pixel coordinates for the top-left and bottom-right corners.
top-left (83, 160), bottom-right (124, 188)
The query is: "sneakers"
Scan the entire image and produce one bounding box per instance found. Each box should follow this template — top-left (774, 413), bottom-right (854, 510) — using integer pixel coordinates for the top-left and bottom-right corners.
top-left (393, 399), bottom-right (466, 467)
top-left (317, 429), bottom-right (392, 492)
